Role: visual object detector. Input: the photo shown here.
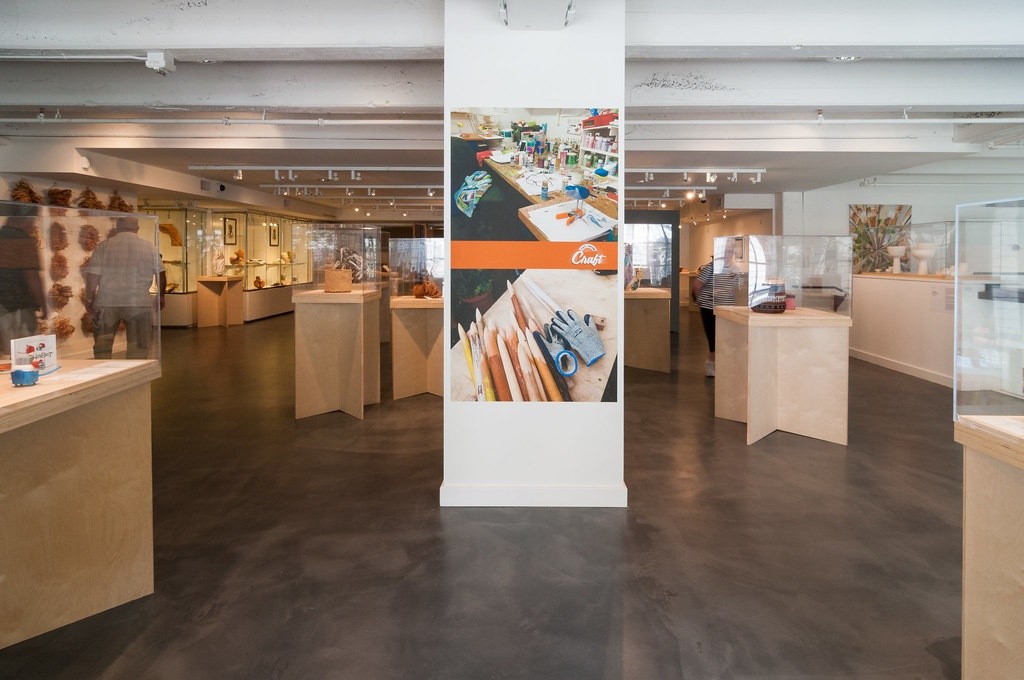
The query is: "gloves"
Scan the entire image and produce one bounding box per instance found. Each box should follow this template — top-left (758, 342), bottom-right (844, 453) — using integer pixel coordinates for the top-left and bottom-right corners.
top-left (533, 323), bottom-right (578, 377)
top-left (551, 309), bottom-right (606, 367)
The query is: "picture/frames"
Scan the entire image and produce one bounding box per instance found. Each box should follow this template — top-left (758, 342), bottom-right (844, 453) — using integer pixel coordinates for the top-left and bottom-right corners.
top-left (269, 224), bottom-right (279, 246)
top-left (224, 218), bottom-right (237, 245)
top-left (849, 205), bottom-right (912, 274)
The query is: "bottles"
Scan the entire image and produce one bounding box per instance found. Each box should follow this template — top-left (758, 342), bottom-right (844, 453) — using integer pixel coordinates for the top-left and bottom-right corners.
top-left (504, 130), bottom-right (618, 205)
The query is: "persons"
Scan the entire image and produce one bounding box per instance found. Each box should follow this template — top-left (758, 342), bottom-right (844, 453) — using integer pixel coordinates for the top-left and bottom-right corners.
top-left (692, 242), bottom-right (742, 377)
top-left (83, 216), bottom-right (166, 360)
top-left (0, 217), bottom-right (49, 356)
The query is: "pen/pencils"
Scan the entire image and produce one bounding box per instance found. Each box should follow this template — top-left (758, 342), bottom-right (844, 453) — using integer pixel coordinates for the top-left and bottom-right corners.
top-left (457, 273), bottom-right (578, 401)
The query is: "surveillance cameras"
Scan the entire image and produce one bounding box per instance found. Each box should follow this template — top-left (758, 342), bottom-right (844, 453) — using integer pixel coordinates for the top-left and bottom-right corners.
top-left (701, 197), bottom-right (707, 204)
top-left (215, 183), bottom-right (226, 193)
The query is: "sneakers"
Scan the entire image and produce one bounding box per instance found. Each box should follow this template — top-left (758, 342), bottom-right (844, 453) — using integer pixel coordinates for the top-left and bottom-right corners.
top-left (704, 358), bottom-right (715, 377)
